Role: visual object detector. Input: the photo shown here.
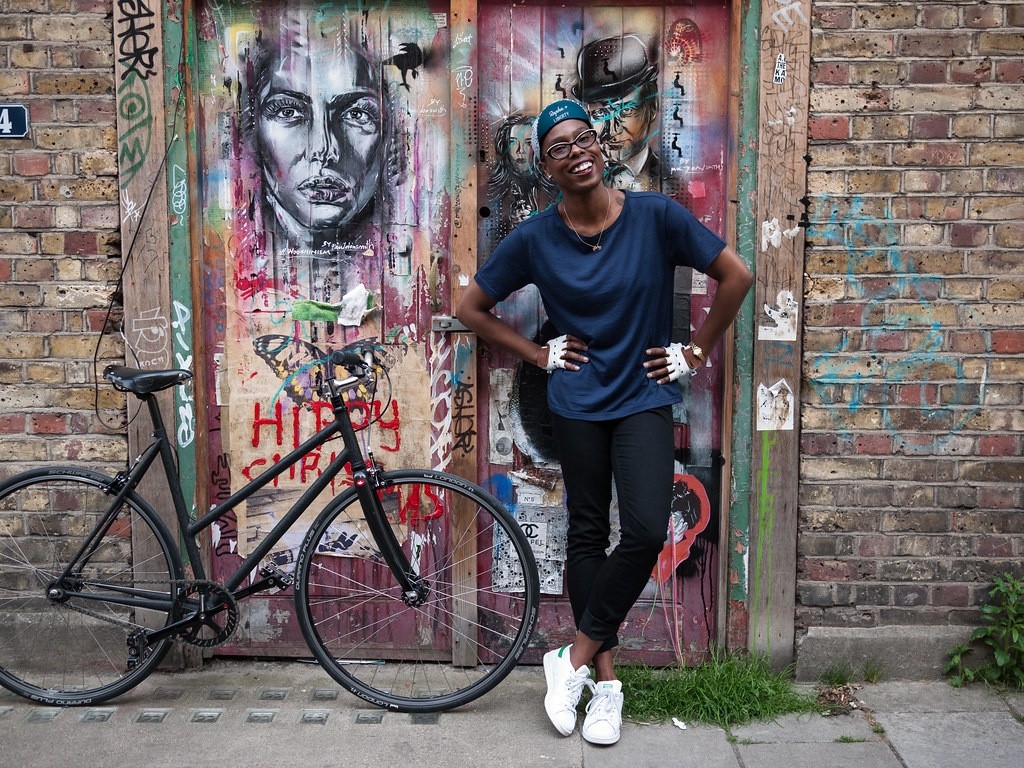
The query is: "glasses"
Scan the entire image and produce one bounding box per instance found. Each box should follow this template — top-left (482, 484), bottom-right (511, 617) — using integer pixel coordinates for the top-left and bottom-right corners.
top-left (541, 129), bottom-right (598, 162)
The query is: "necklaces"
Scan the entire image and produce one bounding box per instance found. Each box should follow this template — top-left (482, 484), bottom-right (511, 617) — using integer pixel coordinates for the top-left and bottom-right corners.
top-left (563, 187), bottom-right (610, 251)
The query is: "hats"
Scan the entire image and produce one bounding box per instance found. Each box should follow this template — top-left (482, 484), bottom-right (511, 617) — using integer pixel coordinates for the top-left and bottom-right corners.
top-left (530, 100), bottom-right (591, 160)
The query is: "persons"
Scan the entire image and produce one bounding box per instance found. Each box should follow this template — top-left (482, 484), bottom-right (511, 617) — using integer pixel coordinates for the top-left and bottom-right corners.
top-left (456, 100), bottom-right (754, 745)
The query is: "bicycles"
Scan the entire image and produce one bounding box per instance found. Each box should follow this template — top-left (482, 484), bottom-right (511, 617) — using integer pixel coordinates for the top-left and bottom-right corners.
top-left (1, 345), bottom-right (540, 712)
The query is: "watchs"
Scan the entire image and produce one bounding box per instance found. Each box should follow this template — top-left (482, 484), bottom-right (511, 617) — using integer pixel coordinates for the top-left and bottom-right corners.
top-left (689, 342), bottom-right (706, 362)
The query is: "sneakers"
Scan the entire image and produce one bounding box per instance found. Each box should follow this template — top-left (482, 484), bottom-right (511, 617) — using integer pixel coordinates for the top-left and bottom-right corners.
top-left (582, 675), bottom-right (625, 745)
top-left (543, 645), bottom-right (591, 736)
